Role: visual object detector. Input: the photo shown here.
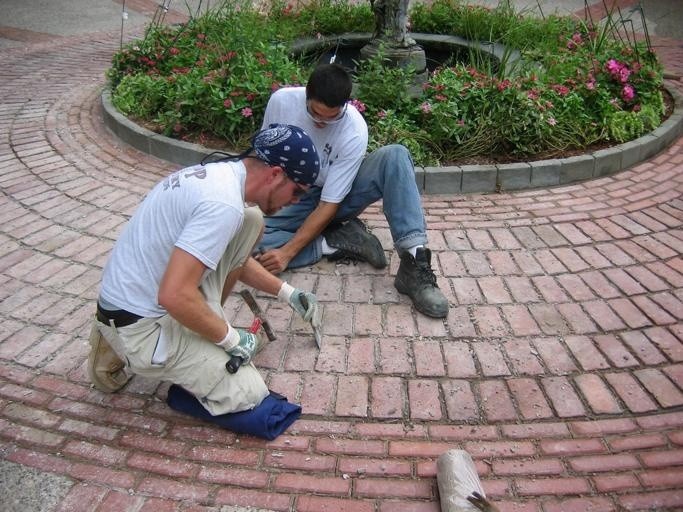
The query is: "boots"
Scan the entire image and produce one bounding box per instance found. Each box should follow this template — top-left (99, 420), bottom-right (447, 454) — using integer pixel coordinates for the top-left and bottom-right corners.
top-left (323, 216), bottom-right (388, 269)
top-left (394, 245), bottom-right (449, 317)
top-left (90, 321), bottom-right (129, 393)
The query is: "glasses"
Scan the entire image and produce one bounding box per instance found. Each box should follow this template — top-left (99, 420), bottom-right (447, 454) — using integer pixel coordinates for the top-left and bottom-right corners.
top-left (282, 172), bottom-right (307, 199)
top-left (305, 101), bottom-right (348, 124)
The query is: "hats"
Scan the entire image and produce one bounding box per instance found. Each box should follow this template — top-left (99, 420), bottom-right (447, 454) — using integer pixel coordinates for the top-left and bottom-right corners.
top-left (247, 122), bottom-right (320, 183)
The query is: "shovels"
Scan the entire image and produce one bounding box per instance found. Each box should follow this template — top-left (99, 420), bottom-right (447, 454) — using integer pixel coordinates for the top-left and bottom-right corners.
top-left (300, 292), bottom-right (324, 352)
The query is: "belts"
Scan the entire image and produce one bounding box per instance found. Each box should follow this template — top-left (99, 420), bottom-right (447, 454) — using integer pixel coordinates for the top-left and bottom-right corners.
top-left (93, 308), bottom-right (140, 328)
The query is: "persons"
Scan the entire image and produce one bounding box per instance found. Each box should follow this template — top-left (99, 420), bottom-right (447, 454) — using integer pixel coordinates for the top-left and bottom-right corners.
top-left (251, 64), bottom-right (449, 319)
top-left (87, 124), bottom-right (323, 416)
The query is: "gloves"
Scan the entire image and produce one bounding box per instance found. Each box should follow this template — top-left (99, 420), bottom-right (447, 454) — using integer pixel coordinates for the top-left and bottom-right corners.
top-left (214, 321), bottom-right (261, 366)
top-left (277, 282), bottom-right (322, 331)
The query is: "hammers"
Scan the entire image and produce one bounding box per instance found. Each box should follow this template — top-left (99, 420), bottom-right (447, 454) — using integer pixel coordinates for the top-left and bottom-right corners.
top-left (225, 290), bottom-right (277, 374)
